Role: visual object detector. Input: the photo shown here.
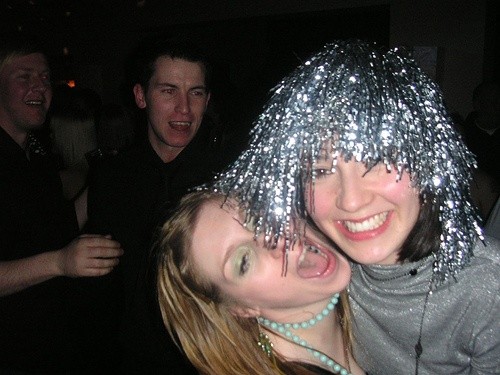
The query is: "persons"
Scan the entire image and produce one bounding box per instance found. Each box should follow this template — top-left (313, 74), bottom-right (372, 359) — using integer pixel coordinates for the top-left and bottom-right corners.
top-left (0, 38), bottom-right (126, 375)
top-left (199, 38), bottom-right (500, 374)
top-left (48, 50), bottom-right (499, 241)
top-left (149, 189), bottom-right (373, 375)
top-left (73, 43), bottom-right (242, 374)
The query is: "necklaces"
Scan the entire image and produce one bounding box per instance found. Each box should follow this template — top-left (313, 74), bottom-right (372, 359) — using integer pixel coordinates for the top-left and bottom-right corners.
top-left (253, 291), bottom-right (350, 375)
top-left (363, 253), bottom-right (431, 281)
